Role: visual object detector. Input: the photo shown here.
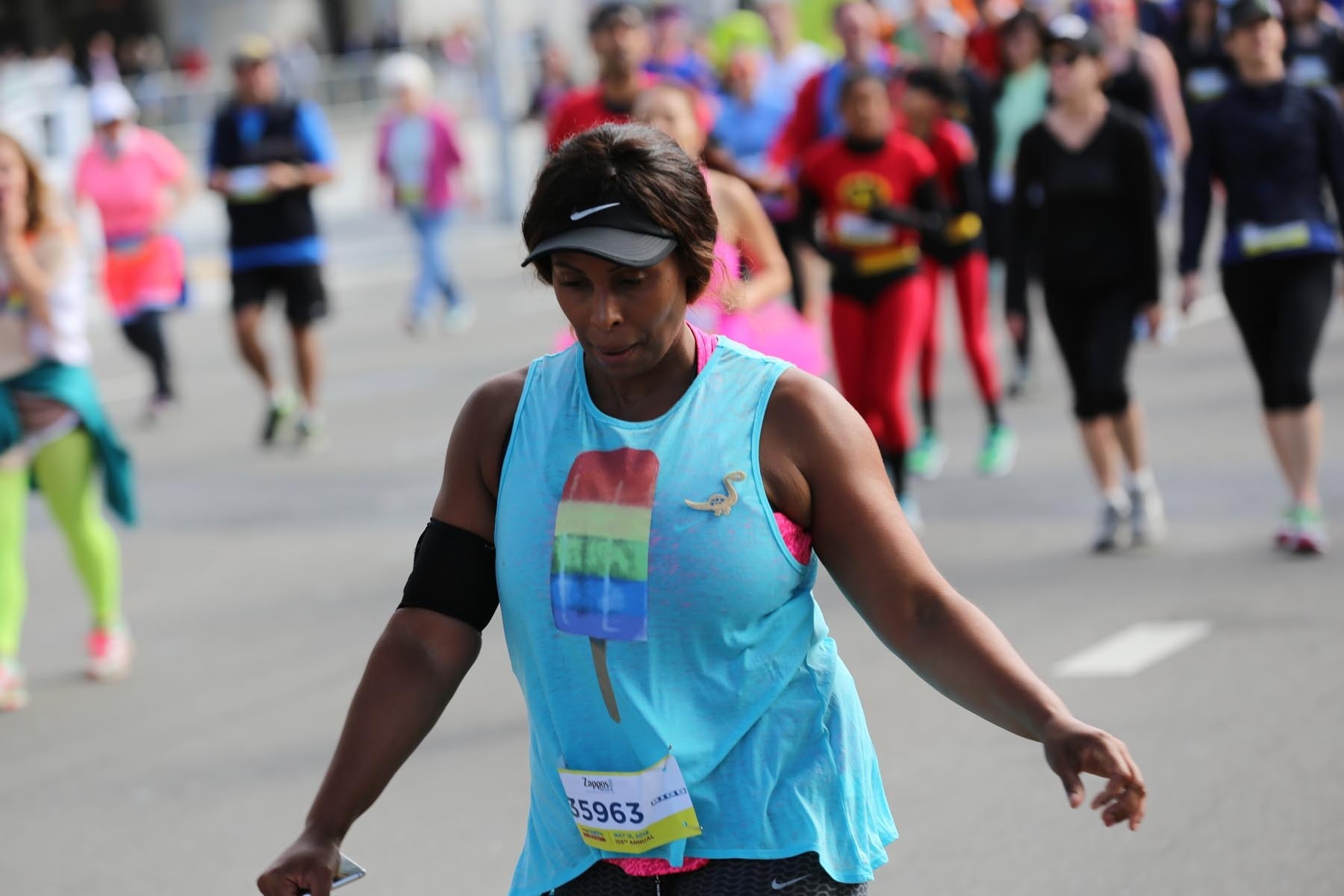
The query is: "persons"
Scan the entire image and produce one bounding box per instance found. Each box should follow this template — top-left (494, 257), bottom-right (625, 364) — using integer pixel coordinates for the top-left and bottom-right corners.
top-left (55, 1), bottom-right (464, 443)
top-left (256, 122), bottom-right (1147, 896)
top-left (515, 3), bottom-right (1344, 555)
top-left (0, 134), bottom-right (134, 710)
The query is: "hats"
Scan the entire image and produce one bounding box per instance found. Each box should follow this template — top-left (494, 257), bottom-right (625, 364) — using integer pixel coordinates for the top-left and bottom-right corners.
top-left (1040, 15), bottom-right (1102, 56)
top-left (1221, 1), bottom-right (1279, 36)
top-left (520, 186), bottom-right (683, 268)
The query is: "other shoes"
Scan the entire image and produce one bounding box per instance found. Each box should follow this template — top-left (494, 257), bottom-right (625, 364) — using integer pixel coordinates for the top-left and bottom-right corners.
top-left (1128, 487), bottom-right (1167, 545)
top-left (976, 425), bottom-right (1003, 475)
top-left (1093, 506), bottom-right (1122, 550)
top-left (1275, 509), bottom-right (1294, 546)
top-left (904, 428), bottom-right (942, 475)
top-left (0, 667), bottom-right (27, 710)
top-left (261, 407), bottom-right (282, 443)
top-left (89, 629), bottom-right (131, 676)
top-left (1294, 510), bottom-right (1326, 554)
